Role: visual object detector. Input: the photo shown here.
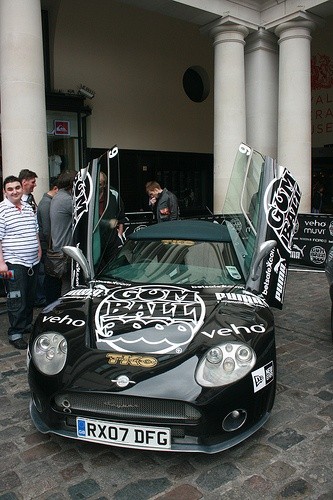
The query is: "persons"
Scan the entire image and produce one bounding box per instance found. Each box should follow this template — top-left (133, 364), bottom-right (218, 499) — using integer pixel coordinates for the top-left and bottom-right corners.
top-left (324, 245), bottom-right (333, 347)
top-left (147, 180), bottom-right (179, 222)
top-left (0, 167), bottom-right (124, 350)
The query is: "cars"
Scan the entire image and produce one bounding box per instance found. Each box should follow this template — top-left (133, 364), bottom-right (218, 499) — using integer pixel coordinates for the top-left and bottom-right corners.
top-left (26, 143), bottom-right (301, 454)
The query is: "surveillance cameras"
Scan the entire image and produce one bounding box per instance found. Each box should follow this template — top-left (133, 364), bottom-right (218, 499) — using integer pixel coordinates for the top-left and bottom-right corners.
top-left (80, 88), bottom-right (94, 100)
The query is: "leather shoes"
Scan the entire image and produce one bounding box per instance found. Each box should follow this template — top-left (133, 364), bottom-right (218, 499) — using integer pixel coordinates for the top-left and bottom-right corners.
top-left (9, 337), bottom-right (27, 349)
top-left (22, 325), bottom-right (32, 333)
